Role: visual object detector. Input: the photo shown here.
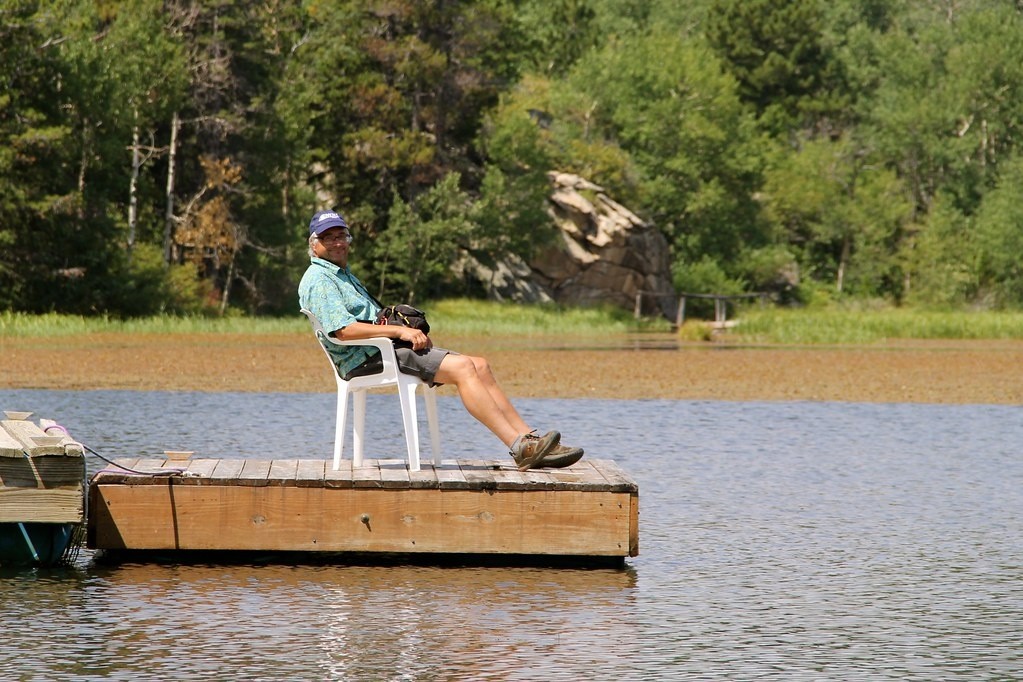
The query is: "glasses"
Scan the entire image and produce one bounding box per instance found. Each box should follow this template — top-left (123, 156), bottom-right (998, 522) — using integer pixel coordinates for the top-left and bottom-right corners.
top-left (314, 234), bottom-right (353, 245)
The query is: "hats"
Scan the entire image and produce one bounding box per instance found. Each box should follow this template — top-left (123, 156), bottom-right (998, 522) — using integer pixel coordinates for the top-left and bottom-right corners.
top-left (306, 210), bottom-right (349, 245)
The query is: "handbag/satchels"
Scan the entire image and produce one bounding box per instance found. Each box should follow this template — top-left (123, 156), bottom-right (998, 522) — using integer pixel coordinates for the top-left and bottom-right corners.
top-left (373, 303), bottom-right (430, 337)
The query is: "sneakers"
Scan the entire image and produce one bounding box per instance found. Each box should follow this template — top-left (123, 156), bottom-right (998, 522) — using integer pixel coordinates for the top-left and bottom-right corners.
top-left (529, 441), bottom-right (584, 469)
top-left (509, 430), bottom-right (561, 472)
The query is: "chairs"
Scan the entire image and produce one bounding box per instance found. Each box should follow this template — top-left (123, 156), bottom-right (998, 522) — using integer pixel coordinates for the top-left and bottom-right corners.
top-left (298, 309), bottom-right (445, 472)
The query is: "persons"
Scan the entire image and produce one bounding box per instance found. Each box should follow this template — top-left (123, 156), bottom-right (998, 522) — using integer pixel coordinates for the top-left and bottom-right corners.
top-left (296, 210), bottom-right (585, 473)
